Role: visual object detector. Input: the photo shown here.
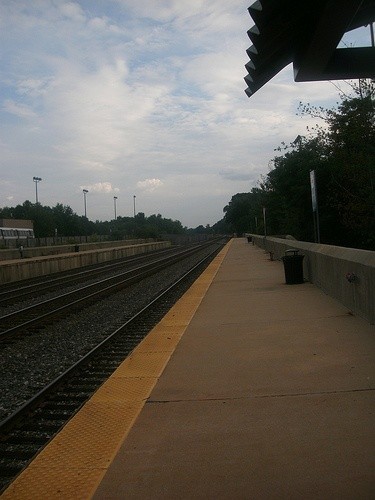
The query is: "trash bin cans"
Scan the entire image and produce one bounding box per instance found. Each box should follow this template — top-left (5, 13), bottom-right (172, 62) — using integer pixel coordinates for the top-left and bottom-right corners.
top-left (281, 249), bottom-right (304, 284)
top-left (247, 237), bottom-right (252, 242)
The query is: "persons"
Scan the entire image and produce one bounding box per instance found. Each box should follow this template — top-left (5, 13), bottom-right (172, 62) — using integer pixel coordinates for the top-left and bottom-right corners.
top-left (19, 245), bottom-right (24, 259)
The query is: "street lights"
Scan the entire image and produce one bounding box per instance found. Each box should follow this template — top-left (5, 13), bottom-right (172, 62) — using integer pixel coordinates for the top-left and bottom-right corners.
top-left (133, 195), bottom-right (136, 216)
top-left (33, 176), bottom-right (42, 202)
top-left (113, 196), bottom-right (117, 219)
top-left (83, 189), bottom-right (89, 217)
